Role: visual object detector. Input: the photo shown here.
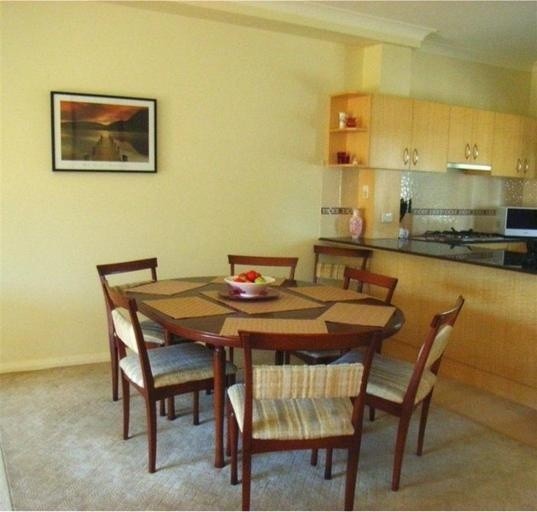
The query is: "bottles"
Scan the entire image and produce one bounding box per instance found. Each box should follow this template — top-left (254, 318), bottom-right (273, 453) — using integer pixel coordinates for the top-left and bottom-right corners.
top-left (399, 226), bottom-right (410, 240)
top-left (347, 208), bottom-right (364, 240)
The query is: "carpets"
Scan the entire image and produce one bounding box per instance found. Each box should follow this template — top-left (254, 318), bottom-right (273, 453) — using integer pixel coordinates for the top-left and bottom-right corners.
top-left (0, 369), bottom-right (537, 511)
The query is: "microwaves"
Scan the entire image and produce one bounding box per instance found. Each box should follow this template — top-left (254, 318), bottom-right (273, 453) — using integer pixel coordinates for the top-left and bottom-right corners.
top-left (495, 205), bottom-right (537, 238)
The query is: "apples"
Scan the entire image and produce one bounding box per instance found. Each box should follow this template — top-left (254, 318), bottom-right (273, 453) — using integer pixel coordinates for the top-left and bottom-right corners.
top-left (234, 270), bottom-right (266, 284)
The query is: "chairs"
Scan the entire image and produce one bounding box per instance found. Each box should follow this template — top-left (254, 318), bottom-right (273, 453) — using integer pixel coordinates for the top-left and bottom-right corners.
top-left (104, 279), bottom-right (238, 473)
top-left (312, 295), bottom-right (465, 491)
top-left (285, 266), bottom-right (398, 421)
top-left (96, 257), bottom-right (212, 416)
top-left (228, 254), bottom-right (299, 385)
top-left (226, 328), bottom-right (384, 511)
top-left (313, 245), bottom-right (370, 293)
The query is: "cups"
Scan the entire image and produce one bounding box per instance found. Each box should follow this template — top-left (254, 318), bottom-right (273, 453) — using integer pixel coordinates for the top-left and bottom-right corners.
top-left (337, 152), bottom-right (349, 163)
top-left (339, 112), bottom-right (361, 129)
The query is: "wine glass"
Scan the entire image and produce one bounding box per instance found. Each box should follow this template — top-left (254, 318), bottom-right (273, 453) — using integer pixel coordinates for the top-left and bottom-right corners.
top-left (223, 275), bottom-right (277, 296)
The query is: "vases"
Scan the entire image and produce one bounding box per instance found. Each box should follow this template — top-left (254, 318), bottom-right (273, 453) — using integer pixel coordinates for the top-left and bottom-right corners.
top-left (349, 209), bottom-right (363, 240)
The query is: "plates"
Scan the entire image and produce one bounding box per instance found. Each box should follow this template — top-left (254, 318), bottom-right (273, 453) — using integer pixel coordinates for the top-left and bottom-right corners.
top-left (217, 290), bottom-right (279, 302)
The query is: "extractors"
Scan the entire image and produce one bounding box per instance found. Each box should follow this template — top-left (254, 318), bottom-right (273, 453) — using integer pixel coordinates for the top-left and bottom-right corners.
top-left (447, 163), bottom-right (491, 173)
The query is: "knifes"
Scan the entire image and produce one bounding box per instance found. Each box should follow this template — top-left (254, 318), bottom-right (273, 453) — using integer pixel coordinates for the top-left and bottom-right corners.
top-left (399, 198), bottom-right (411, 222)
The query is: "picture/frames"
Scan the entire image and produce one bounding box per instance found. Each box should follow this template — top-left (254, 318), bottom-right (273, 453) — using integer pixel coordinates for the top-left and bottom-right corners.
top-left (50, 91), bottom-right (157, 172)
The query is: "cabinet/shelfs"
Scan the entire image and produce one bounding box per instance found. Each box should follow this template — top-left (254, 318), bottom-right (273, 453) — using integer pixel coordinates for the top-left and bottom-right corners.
top-left (448, 105), bottom-right (493, 171)
top-left (368, 93), bottom-right (447, 173)
top-left (465, 111), bottom-right (537, 179)
top-left (326, 92), bottom-right (369, 169)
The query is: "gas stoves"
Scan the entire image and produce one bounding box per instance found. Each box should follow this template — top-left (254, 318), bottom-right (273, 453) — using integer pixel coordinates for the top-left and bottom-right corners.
top-left (416, 228), bottom-right (504, 241)
